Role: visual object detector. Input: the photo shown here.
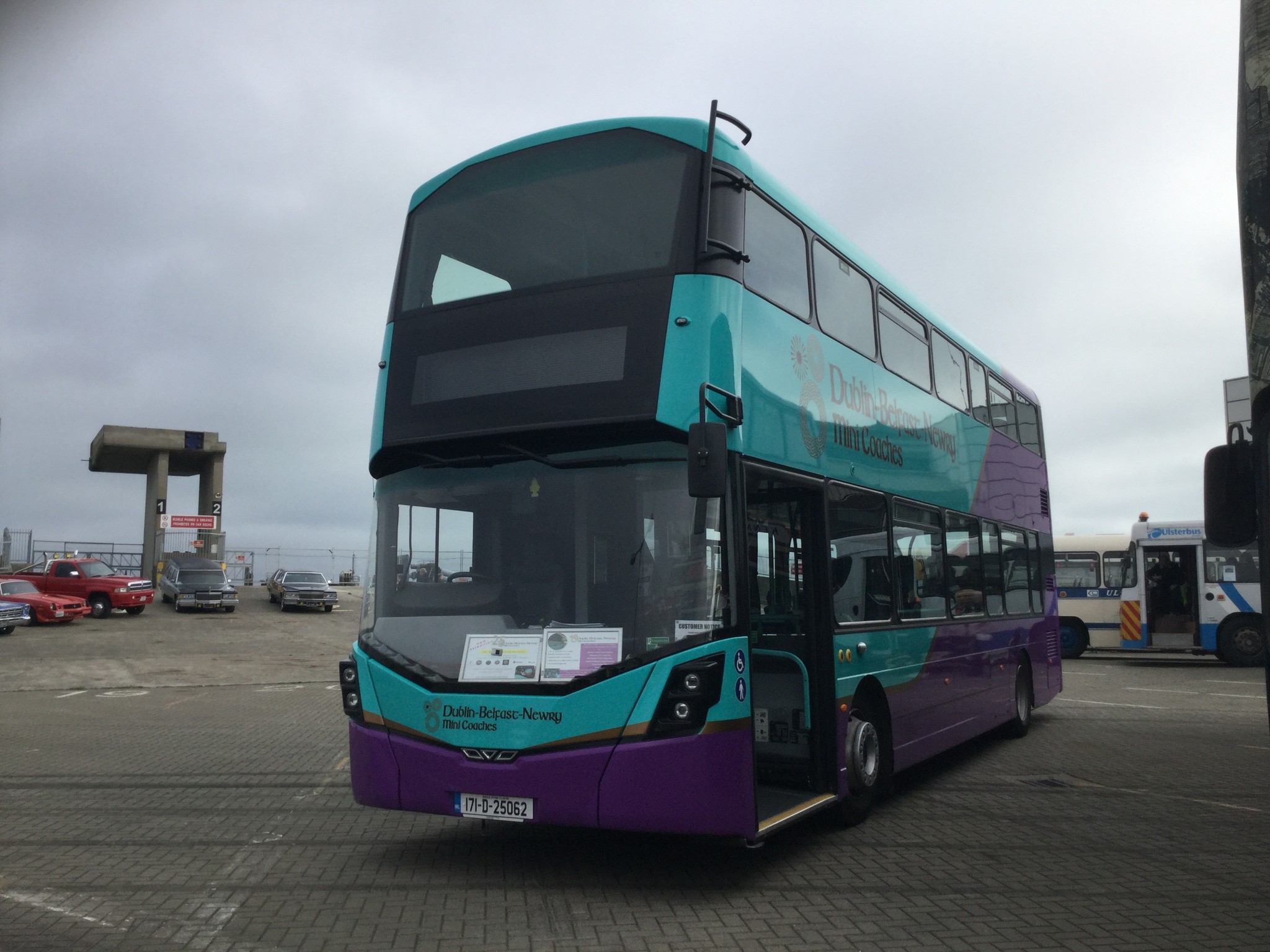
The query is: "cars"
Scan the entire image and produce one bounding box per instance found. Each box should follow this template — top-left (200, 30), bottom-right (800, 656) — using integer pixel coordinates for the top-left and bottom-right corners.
top-left (265, 568), bottom-right (339, 613)
top-left (0, 578), bottom-right (92, 627)
top-left (0, 600), bottom-right (31, 635)
top-left (158, 557), bottom-right (239, 613)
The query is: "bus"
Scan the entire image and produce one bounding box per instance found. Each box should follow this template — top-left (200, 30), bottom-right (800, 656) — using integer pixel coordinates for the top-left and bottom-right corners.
top-left (1052, 510), bottom-right (1268, 669)
top-left (337, 98), bottom-right (1064, 846)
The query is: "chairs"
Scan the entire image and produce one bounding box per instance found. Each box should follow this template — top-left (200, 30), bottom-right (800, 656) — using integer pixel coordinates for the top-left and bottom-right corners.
top-left (1226, 552), bottom-right (1260, 583)
top-left (1106, 574), bottom-right (1113, 587)
top-left (1073, 575), bottom-right (1083, 587)
top-left (827, 546), bottom-right (1050, 628)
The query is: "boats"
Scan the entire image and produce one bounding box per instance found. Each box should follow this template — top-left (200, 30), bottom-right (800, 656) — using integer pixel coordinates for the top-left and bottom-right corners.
top-left (339, 552), bottom-right (361, 585)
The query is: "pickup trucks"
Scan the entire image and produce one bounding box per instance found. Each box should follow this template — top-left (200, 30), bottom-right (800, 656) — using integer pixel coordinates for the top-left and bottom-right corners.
top-left (0, 549), bottom-right (155, 619)
